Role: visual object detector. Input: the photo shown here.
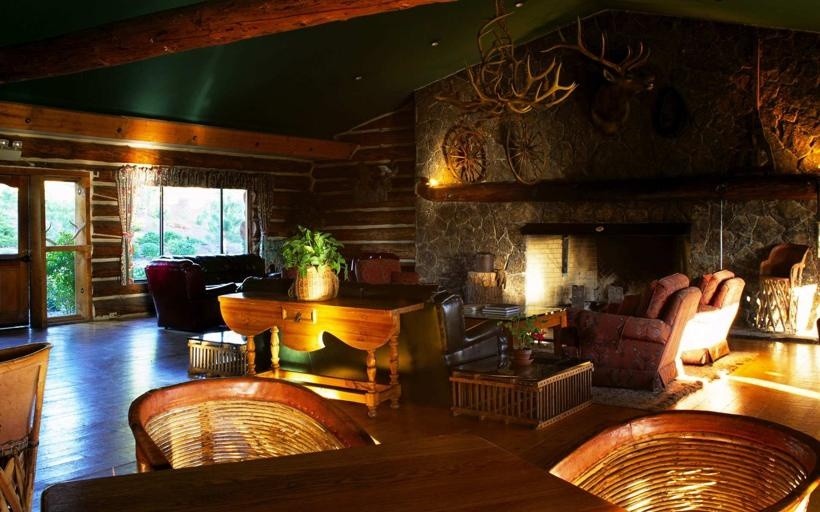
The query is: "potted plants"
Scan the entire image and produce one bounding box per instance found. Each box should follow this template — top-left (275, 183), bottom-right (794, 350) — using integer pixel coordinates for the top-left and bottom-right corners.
top-left (280, 228), bottom-right (351, 302)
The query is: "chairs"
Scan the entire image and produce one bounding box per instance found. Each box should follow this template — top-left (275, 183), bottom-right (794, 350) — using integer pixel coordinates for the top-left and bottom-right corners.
top-left (760, 242), bottom-right (810, 288)
top-left (548, 410), bottom-right (820, 512)
top-left (0, 342), bottom-right (55, 512)
top-left (128, 376), bottom-right (375, 478)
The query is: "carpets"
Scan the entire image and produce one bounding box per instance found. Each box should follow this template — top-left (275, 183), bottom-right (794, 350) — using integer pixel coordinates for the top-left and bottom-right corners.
top-left (586, 337), bottom-right (703, 417)
top-left (682, 350), bottom-right (760, 381)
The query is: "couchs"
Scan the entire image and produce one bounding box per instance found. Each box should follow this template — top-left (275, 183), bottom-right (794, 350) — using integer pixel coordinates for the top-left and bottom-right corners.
top-left (234, 275), bottom-right (511, 406)
top-left (682, 276), bottom-right (746, 366)
top-left (173, 253), bottom-right (266, 285)
top-left (144, 257), bottom-right (237, 334)
top-left (574, 287), bottom-right (702, 391)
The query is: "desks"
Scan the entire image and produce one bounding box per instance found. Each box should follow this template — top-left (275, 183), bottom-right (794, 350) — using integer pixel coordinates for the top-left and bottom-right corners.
top-left (448, 352), bottom-right (594, 431)
top-left (187, 330), bottom-right (272, 379)
top-left (463, 307), bottom-right (569, 352)
top-left (217, 291), bottom-right (425, 420)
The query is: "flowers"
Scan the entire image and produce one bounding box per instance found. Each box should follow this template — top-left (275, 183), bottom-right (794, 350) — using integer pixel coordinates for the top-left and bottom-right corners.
top-left (497, 312), bottom-right (545, 350)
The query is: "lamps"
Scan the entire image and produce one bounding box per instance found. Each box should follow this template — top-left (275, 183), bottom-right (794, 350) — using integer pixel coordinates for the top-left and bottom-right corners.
top-left (0, 138), bottom-right (22, 161)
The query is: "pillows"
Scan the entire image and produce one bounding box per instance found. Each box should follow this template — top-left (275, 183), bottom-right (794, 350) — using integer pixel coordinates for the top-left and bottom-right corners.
top-left (642, 273), bottom-right (689, 320)
top-left (699, 269), bottom-right (736, 304)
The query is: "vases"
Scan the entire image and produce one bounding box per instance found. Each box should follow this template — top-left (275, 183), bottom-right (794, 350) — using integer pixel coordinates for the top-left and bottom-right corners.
top-left (514, 349), bottom-right (532, 366)
